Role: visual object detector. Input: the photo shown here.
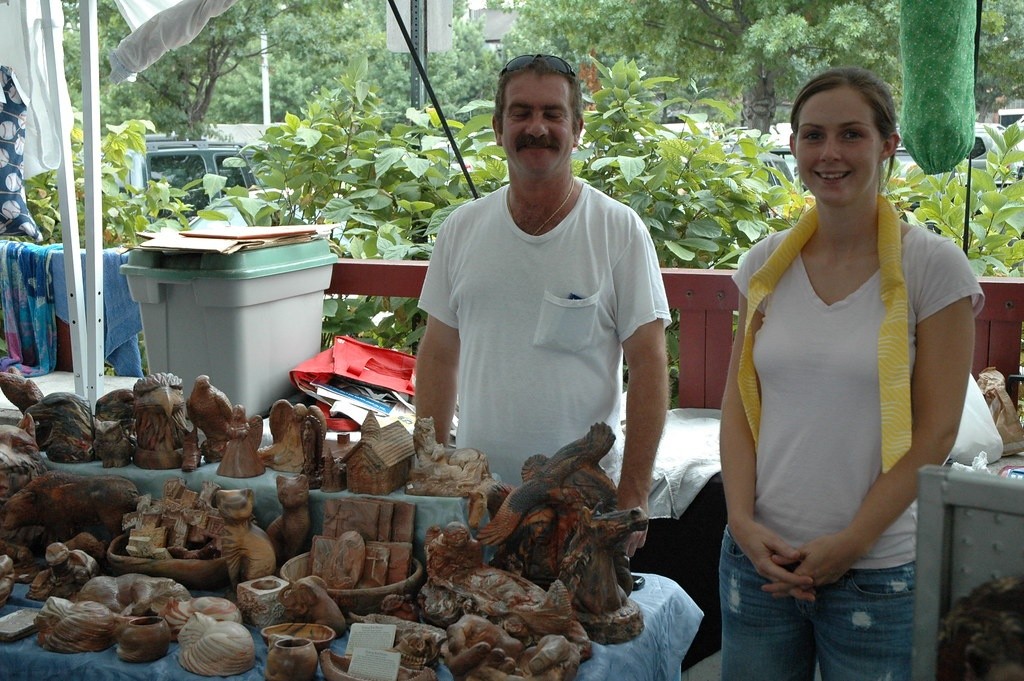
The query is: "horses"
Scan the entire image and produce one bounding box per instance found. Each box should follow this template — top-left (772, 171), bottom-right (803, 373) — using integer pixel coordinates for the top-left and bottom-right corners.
top-left (560, 502), bottom-right (648, 613)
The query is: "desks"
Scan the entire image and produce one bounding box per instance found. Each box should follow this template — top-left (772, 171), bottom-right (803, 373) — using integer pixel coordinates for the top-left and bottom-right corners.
top-left (0, 449), bottom-right (708, 681)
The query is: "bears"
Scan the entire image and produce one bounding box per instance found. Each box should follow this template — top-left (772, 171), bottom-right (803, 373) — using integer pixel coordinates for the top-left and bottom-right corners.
top-left (3, 471), bottom-right (141, 539)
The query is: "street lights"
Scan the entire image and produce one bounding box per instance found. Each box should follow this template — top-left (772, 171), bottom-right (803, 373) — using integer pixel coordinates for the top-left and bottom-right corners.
top-left (261, 5), bottom-right (286, 125)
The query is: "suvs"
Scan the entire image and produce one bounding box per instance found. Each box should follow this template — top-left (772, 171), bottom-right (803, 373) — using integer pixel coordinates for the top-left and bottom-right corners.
top-left (79, 134), bottom-right (287, 228)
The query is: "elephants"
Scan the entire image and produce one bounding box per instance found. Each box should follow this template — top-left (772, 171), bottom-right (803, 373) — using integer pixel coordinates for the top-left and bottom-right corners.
top-left (278, 576), bottom-right (347, 637)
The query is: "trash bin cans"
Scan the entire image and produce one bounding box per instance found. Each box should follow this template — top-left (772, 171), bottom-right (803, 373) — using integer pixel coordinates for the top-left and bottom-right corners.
top-left (119, 239), bottom-right (339, 420)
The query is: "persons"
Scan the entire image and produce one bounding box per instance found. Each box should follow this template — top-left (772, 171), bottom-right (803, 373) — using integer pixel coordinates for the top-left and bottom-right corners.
top-left (719, 66), bottom-right (986, 681)
top-left (416, 54), bottom-right (673, 558)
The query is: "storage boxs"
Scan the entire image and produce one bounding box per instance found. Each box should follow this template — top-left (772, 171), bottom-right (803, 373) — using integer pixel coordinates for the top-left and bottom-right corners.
top-left (120, 239), bottom-right (340, 423)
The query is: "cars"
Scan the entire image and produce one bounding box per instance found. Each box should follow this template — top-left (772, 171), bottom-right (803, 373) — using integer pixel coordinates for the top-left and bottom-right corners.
top-left (625, 108), bottom-right (1024, 219)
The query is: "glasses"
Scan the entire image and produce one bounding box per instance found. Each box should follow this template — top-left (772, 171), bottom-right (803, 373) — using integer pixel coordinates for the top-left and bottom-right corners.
top-left (499, 54), bottom-right (575, 78)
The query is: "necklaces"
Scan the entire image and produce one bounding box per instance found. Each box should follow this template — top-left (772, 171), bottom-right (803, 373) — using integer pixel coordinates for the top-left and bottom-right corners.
top-left (507, 174), bottom-right (575, 236)
